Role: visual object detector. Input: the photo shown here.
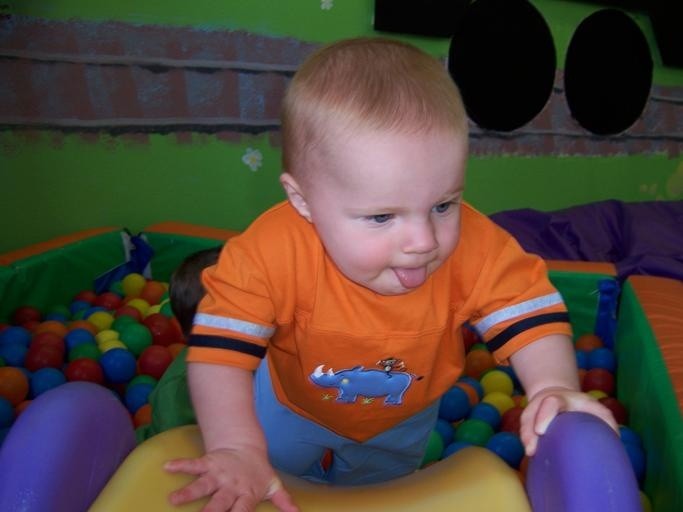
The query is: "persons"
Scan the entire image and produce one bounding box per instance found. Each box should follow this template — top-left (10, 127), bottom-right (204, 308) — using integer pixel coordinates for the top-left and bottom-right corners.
top-left (155, 35), bottom-right (626, 510)
top-left (131, 242), bottom-right (226, 447)
